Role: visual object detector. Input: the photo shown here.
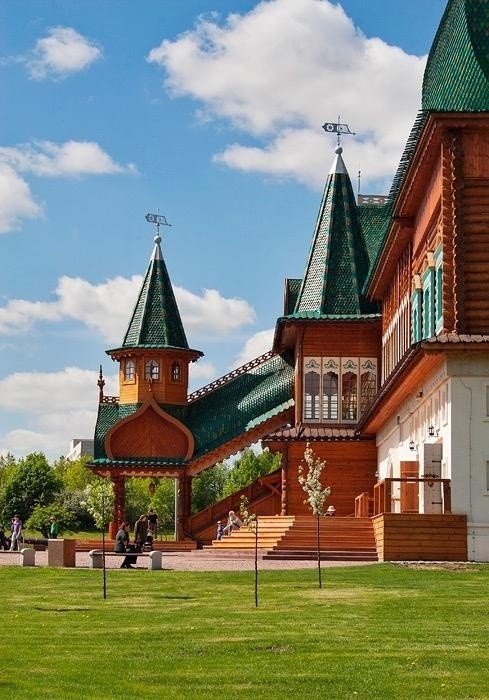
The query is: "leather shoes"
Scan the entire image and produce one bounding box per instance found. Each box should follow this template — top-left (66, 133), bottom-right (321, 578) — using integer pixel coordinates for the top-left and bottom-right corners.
top-left (120, 564), bottom-right (134, 568)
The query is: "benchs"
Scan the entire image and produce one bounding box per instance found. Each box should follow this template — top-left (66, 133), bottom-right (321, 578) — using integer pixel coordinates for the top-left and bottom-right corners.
top-left (0, 547), bottom-right (35, 567)
top-left (89, 548), bottom-right (163, 570)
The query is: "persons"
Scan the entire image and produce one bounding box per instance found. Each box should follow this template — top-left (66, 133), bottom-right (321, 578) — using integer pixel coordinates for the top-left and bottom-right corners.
top-left (114, 521), bottom-right (139, 569)
top-left (222, 511), bottom-right (243, 536)
top-left (49, 515), bottom-right (59, 539)
top-left (8, 514), bottom-right (22, 552)
top-left (0, 518), bottom-right (18, 551)
top-left (216, 521), bottom-right (224, 541)
top-left (134, 513), bottom-right (148, 549)
top-left (324, 506), bottom-right (337, 517)
top-left (148, 508), bottom-right (158, 542)
